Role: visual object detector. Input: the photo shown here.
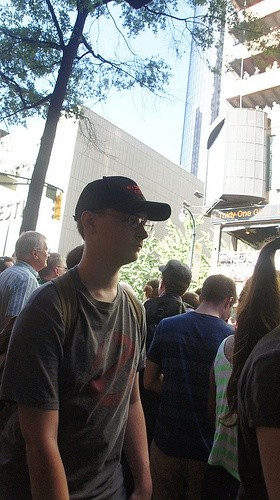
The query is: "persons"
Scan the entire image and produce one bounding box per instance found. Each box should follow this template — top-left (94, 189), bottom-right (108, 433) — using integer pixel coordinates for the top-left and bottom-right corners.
top-left (226, 237), bottom-right (280, 500)
top-left (142, 279), bottom-right (241, 327)
top-left (205, 271), bottom-right (280, 500)
top-left (0, 244), bottom-right (84, 285)
top-left (143, 274), bottom-right (237, 500)
top-left (0, 230), bottom-right (51, 371)
top-left (118, 259), bottom-right (195, 500)
top-left (0, 174), bottom-right (173, 500)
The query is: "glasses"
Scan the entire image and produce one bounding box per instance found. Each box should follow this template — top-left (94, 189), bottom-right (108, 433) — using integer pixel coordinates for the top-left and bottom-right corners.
top-left (56, 265), bottom-right (68, 272)
top-left (95, 211), bottom-right (148, 228)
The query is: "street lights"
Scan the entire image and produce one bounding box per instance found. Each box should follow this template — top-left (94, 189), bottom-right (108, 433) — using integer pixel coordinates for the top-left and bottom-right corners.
top-left (179, 206), bottom-right (195, 268)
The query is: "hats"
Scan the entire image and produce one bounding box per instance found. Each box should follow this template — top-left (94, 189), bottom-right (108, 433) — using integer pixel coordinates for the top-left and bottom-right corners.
top-left (159, 260), bottom-right (192, 291)
top-left (73, 175), bottom-right (171, 222)
